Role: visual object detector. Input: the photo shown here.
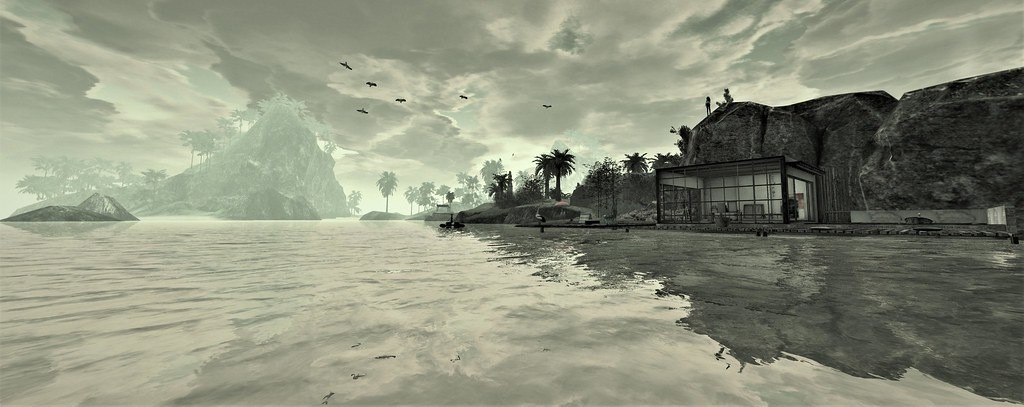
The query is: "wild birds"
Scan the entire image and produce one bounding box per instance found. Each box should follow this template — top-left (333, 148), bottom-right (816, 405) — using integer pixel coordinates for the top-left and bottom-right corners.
top-left (366, 82), bottom-right (377, 87)
top-left (395, 99), bottom-right (406, 103)
top-left (459, 95), bottom-right (467, 99)
top-left (543, 104), bottom-right (552, 109)
top-left (340, 62), bottom-right (352, 70)
top-left (356, 108), bottom-right (368, 114)
